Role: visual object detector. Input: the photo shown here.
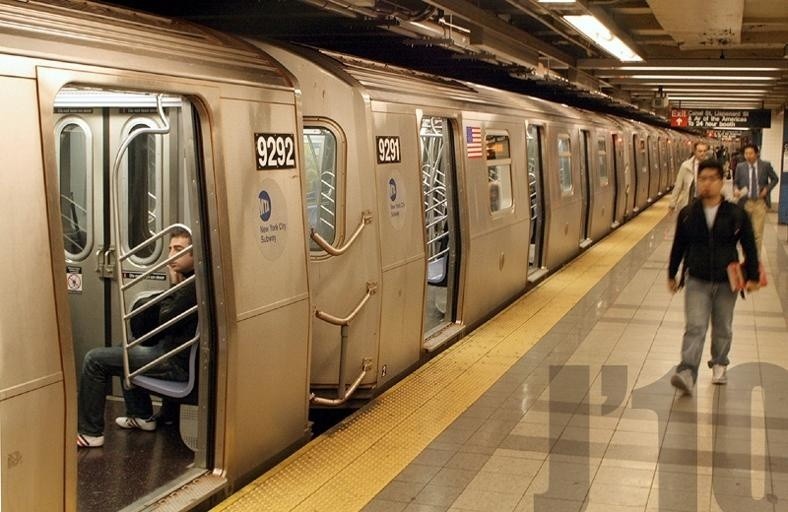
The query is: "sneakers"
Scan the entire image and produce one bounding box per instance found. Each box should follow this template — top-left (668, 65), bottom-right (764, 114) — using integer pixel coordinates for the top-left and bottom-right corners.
top-left (670, 369), bottom-right (694, 395)
top-left (711, 364), bottom-right (728, 385)
top-left (115, 416), bottom-right (157, 431)
top-left (76, 433), bottom-right (105, 448)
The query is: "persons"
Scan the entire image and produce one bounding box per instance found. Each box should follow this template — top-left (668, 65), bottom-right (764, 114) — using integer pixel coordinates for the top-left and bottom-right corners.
top-left (77, 226), bottom-right (198, 449)
top-left (668, 139), bottom-right (779, 259)
top-left (666, 158), bottom-right (760, 398)
top-left (490, 184), bottom-right (499, 212)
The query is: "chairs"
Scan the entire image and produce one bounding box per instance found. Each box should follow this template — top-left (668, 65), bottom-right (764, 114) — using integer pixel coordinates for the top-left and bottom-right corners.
top-left (428, 252), bottom-right (449, 288)
top-left (132, 320), bottom-right (199, 429)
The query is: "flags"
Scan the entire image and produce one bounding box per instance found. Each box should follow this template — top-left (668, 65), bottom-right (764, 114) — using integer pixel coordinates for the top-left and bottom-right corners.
top-left (465, 126), bottom-right (482, 158)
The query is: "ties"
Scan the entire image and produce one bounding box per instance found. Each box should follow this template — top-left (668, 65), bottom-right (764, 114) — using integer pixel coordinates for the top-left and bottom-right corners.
top-left (751, 165), bottom-right (758, 203)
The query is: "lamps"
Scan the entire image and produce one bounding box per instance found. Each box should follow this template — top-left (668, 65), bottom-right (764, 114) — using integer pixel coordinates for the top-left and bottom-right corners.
top-left (563, 5), bottom-right (648, 65)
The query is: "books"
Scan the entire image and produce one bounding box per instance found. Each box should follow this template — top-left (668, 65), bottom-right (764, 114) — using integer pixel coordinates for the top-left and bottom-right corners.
top-left (726, 261), bottom-right (768, 293)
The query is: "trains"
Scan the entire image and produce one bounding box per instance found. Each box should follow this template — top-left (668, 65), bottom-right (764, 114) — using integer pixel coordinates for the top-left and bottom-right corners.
top-left (0, 0), bottom-right (726, 512)
